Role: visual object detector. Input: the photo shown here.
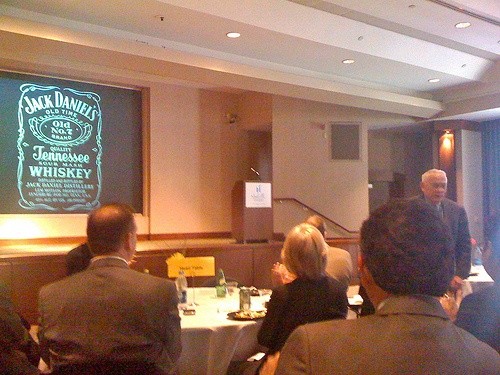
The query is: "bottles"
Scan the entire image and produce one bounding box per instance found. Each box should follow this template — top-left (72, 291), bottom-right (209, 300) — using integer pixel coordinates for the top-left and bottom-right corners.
top-left (216, 268), bottom-right (227, 297)
top-left (144, 268), bottom-right (149, 274)
top-left (175, 271), bottom-right (188, 309)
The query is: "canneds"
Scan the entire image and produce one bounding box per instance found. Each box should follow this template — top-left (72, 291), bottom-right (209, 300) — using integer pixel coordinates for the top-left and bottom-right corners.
top-left (239, 288), bottom-right (250, 311)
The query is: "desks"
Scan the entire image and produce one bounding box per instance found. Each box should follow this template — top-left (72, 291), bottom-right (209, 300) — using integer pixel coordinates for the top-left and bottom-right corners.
top-left (174, 286), bottom-right (273, 375)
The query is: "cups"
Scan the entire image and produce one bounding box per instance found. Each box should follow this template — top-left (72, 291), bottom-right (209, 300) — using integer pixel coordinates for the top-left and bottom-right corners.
top-left (227, 281), bottom-right (238, 296)
top-left (240, 286), bottom-right (250, 312)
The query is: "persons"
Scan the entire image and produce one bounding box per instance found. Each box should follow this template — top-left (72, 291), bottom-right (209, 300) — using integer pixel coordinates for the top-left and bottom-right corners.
top-left (307, 215), bottom-right (352, 290)
top-left (65, 243), bottom-right (91, 274)
top-left (38, 202), bottom-right (181, 375)
top-left (258, 222), bottom-right (349, 353)
top-left (0, 296), bottom-right (40, 375)
top-left (413, 168), bottom-right (471, 317)
top-left (257, 200), bottom-right (500, 375)
top-left (454, 212), bottom-right (500, 355)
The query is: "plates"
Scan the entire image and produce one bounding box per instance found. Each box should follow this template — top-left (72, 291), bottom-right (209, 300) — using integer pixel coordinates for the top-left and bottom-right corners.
top-left (227, 310), bottom-right (268, 319)
top-left (239, 290), bottom-right (269, 295)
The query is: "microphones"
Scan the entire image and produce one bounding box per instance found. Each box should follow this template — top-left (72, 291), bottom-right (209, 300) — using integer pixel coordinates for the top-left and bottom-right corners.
top-left (251, 167), bottom-right (260, 181)
